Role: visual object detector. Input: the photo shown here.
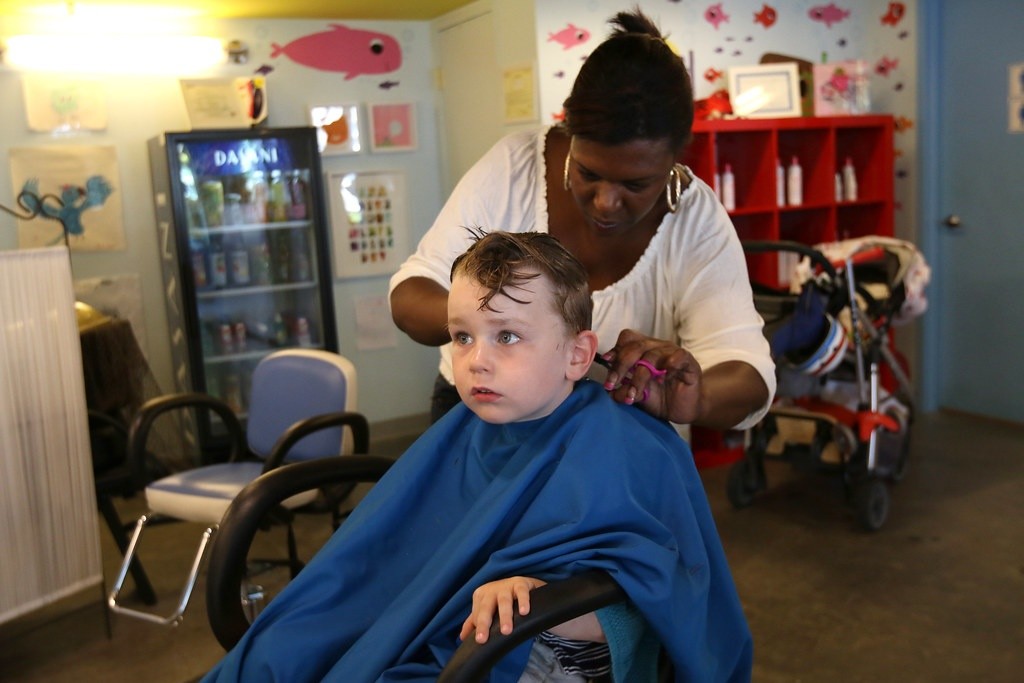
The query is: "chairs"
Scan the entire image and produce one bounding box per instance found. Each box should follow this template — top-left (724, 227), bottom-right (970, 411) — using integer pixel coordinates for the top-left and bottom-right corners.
top-left (108, 347), bottom-right (370, 627)
top-left (206, 415), bottom-right (681, 682)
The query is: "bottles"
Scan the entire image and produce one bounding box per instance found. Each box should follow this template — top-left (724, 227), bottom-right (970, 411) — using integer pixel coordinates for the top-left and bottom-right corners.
top-left (182, 172), bottom-right (325, 420)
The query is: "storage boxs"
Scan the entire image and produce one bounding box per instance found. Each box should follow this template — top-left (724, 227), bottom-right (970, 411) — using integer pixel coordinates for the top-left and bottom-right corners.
top-left (814, 62), bottom-right (871, 118)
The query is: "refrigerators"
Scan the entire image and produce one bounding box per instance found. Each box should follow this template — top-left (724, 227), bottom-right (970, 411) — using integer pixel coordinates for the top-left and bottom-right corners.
top-left (146, 126), bottom-right (339, 471)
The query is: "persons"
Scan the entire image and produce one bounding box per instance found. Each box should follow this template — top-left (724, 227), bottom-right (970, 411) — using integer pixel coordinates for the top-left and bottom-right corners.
top-left (197, 233), bottom-right (754, 683)
top-left (389, 11), bottom-right (776, 443)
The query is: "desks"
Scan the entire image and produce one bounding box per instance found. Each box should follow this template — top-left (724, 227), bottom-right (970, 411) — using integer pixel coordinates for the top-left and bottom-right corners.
top-left (81, 309), bottom-right (164, 603)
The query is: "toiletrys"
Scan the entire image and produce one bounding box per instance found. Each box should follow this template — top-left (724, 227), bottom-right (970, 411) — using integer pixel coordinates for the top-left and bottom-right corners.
top-left (776, 160), bottom-right (786, 207)
top-left (787, 157), bottom-right (803, 206)
top-left (723, 164), bottom-right (735, 211)
top-left (835, 169), bottom-right (843, 202)
top-left (714, 168), bottom-right (720, 201)
top-left (843, 158), bottom-right (858, 202)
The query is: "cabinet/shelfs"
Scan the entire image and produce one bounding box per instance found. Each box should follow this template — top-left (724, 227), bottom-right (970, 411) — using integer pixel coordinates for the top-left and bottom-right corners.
top-left (673, 112), bottom-right (903, 470)
